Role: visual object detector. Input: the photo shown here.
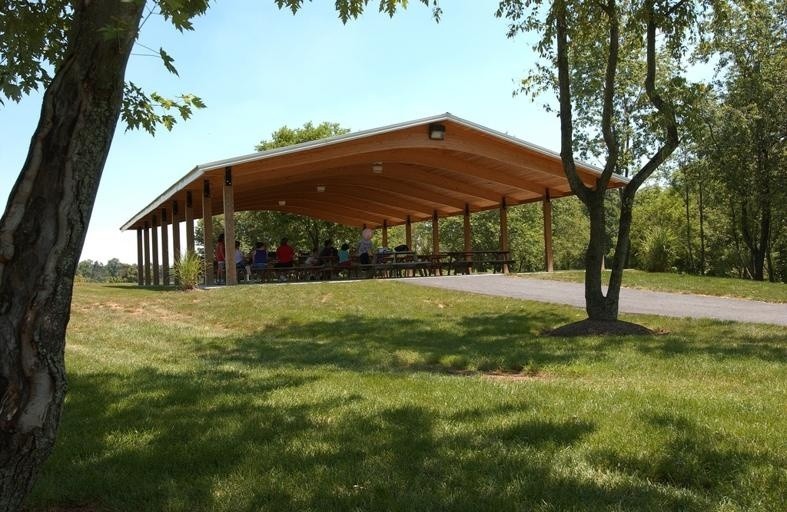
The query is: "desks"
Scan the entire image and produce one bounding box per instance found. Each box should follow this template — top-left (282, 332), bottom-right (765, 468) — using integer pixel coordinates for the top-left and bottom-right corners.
top-left (443, 249), bottom-right (510, 275)
top-left (373, 251), bottom-right (423, 278)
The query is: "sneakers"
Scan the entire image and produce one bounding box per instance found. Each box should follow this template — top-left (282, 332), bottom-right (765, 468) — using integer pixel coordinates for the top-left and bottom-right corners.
top-left (215, 276), bottom-right (290, 285)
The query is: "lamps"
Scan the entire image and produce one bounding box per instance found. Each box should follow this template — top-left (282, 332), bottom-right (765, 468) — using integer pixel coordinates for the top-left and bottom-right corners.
top-left (429, 124), bottom-right (445, 139)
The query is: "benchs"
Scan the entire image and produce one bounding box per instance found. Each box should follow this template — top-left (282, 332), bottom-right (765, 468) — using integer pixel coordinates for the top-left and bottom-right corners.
top-left (221, 259), bottom-right (516, 282)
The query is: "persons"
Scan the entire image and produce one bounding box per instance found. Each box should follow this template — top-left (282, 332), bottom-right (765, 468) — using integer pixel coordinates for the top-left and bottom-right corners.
top-left (274, 237), bottom-right (294, 282)
top-left (252, 241), bottom-right (272, 283)
top-left (303, 248), bottom-right (319, 280)
top-left (337, 244), bottom-right (353, 280)
top-left (235, 241), bottom-right (248, 285)
top-left (351, 228), bottom-right (374, 264)
top-left (216, 233), bottom-right (225, 284)
top-left (318, 239), bottom-right (338, 280)
